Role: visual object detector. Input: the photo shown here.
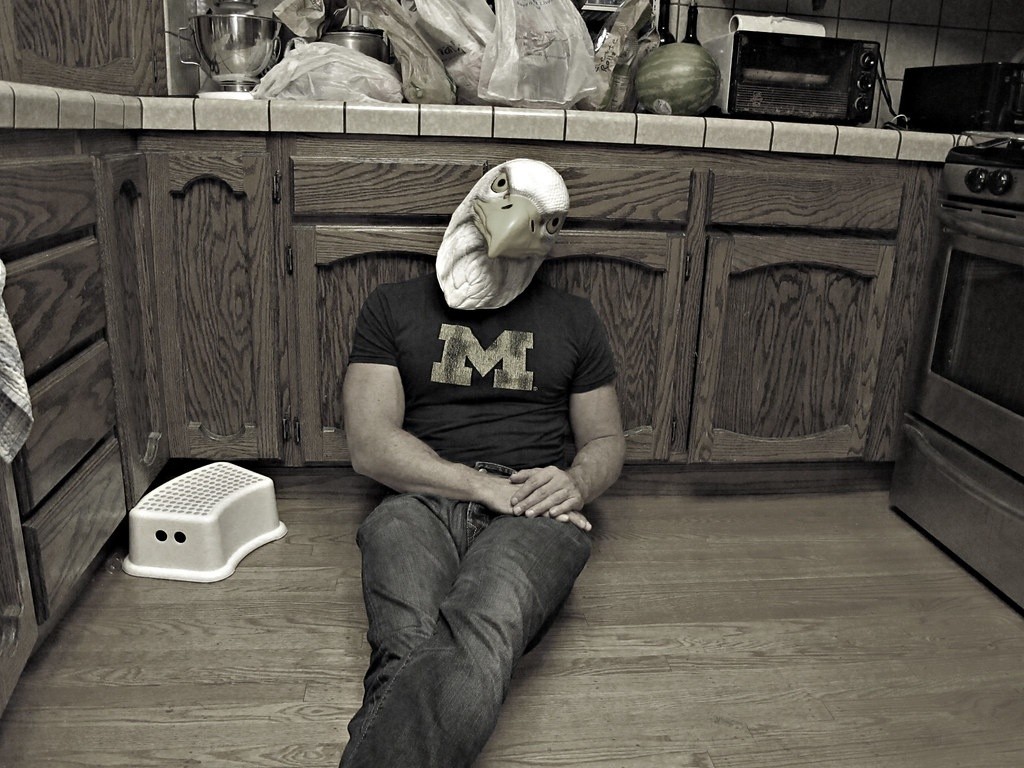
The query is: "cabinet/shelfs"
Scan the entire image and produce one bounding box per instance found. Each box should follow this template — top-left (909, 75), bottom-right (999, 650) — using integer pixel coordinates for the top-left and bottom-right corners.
top-left (681, 167), bottom-right (904, 466)
top-left (291, 154), bottom-right (695, 466)
top-left (0, 146), bottom-right (290, 717)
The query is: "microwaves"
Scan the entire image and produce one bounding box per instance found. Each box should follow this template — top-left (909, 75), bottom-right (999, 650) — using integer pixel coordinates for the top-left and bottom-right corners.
top-left (702, 29), bottom-right (881, 125)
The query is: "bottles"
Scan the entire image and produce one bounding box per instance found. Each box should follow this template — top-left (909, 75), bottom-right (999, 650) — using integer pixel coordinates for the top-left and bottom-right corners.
top-left (681, 0), bottom-right (701, 45)
top-left (654, 0), bottom-right (676, 47)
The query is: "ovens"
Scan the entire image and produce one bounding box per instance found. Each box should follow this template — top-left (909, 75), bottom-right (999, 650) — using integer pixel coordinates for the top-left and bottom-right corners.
top-left (890, 198), bottom-right (1024, 612)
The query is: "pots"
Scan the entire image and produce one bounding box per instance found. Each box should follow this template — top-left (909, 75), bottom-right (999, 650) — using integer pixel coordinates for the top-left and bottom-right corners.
top-left (178, 14), bottom-right (287, 83)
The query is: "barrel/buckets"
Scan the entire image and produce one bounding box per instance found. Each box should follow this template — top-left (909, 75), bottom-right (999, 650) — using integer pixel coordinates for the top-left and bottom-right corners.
top-left (319, 32), bottom-right (387, 64)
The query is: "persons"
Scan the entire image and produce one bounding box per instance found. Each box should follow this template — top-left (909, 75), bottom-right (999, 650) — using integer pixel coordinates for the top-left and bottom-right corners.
top-left (340, 157), bottom-right (625, 768)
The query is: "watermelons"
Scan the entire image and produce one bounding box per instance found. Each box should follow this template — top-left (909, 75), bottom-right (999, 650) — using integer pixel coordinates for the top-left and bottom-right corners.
top-left (634, 43), bottom-right (721, 115)
